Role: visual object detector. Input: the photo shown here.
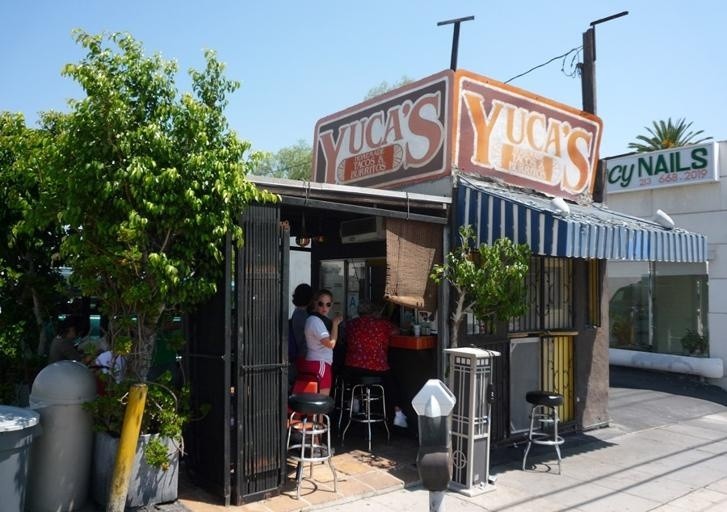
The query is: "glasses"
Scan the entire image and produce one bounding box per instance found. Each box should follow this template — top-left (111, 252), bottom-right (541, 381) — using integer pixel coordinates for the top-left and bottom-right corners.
top-left (316, 300), bottom-right (334, 307)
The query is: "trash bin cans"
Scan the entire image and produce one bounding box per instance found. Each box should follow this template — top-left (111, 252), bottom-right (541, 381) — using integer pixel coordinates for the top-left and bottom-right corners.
top-left (30, 360), bottom-right (99, 512)
top-left (0, 406), bottom-right (41, 512)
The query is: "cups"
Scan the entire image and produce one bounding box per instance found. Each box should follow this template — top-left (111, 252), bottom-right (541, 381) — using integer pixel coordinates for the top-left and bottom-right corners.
top-left (414, 324), bottom-right (421, 337)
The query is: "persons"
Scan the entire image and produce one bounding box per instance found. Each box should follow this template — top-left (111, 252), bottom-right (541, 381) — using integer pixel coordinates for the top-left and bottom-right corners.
top-left (340, 298), bottom-right (410, 430)
top-left (47, 323), bottom-right (77, 361)
top-left (147, 354), bottom-right (194, 476)
top-left (294, 289), bottom-right (343, 457)
top-left (291, 283), bottom-right (312, 351)
top-left (92, 344), bottom-right (126, 384)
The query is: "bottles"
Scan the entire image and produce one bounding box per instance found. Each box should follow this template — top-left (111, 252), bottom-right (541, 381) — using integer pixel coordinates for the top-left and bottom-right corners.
top-left (349, 297), bottom-right (355, 311)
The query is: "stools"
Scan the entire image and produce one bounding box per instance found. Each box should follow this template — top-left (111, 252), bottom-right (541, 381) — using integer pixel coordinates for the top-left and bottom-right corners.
top-left (522, 389), bottom-right (563, 474)
top-left (336, 368), bottom-right (394, 453)
top-left (286, 390), bottom-right (338, 497)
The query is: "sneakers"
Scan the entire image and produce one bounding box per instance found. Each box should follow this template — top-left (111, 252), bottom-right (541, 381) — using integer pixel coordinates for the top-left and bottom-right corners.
top-left (313, 443), bottom-right (336, 458)
top-left (394, 416), bottom-right (408, 429)
